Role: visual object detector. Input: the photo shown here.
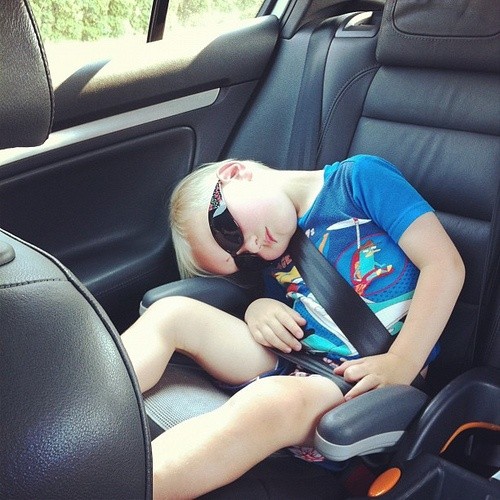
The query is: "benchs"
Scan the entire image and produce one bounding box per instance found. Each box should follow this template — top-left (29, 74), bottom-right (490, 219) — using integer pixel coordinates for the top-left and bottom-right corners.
top-left (138, 0), bottom-right (500, 500)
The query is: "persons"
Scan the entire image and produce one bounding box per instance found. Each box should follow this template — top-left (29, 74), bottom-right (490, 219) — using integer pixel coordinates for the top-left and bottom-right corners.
top-left (119, 154), bottom-right (466, 500)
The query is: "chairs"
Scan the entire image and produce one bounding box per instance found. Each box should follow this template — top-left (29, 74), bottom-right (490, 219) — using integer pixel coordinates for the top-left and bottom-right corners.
top-left (0, 0), bottom-right (153, 500)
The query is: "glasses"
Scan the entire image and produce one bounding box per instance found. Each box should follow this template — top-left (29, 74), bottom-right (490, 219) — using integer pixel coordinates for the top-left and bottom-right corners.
top-left (208, 179), bottom-right (268, 273)
